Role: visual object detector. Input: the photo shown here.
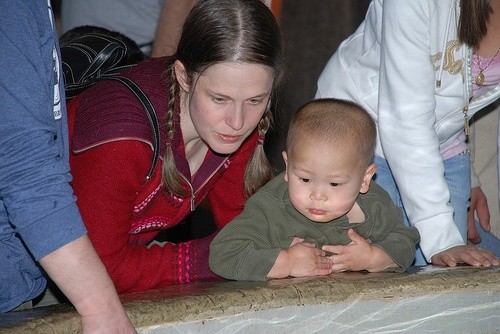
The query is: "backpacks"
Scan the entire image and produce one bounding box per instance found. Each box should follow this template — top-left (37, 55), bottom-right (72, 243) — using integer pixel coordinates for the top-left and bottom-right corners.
top-left (59, 25), bottom-right (160, 186)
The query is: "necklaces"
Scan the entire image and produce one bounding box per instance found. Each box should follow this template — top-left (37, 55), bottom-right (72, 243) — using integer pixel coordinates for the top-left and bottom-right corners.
top-left (473, 37), bottom-right (497, 90)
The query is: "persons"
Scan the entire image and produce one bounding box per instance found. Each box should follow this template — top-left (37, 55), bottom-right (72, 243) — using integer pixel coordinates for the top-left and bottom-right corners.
top-left (1, 2), bottom-right (137, 332)
top-left (314, 2), bottom-right (496, 268)
top-left (48, 0), bottom-right (281, 293)
top-left (207, 97), bottom-right (422, 283)
top-left (52, 0), bottom-right (200, 62)
top-left (466, 96), bottom-right (500, 257)
